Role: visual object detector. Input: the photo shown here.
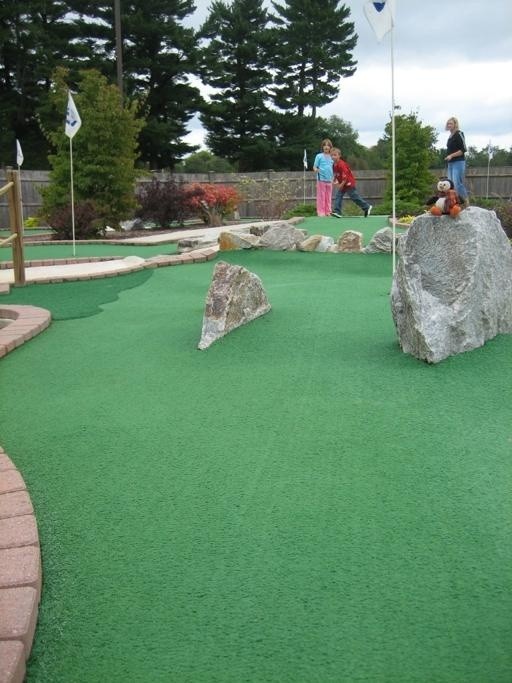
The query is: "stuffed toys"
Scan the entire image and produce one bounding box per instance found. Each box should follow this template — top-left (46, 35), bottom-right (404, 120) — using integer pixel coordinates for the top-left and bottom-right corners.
top-left (426, 175), bottom-right (466, 218)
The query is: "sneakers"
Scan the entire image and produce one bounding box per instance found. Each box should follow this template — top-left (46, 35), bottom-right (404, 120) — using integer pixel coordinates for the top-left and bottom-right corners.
top-left (364, 205), bottom-right (373, 218)
top-left (330, 212), bottom-right (342, 217)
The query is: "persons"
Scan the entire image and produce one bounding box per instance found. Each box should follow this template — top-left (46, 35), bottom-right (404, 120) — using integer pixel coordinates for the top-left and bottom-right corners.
top-left (330, 147), bottom-right (372, 218)
top-left (313, 139), bottom-right (333, 216)
top-left (444, 116), bottom-right (470, 208)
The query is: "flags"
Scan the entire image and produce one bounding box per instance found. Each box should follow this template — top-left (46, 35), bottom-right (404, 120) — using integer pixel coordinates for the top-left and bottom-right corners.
top-left (64, 89), bottom-right (83, 138)
top-left (303, 149), bottom-right (308, 168)
top-left (16, 138), bottom-right (24, 166)
top-left (363, 0), bottom-right (396, 41)
